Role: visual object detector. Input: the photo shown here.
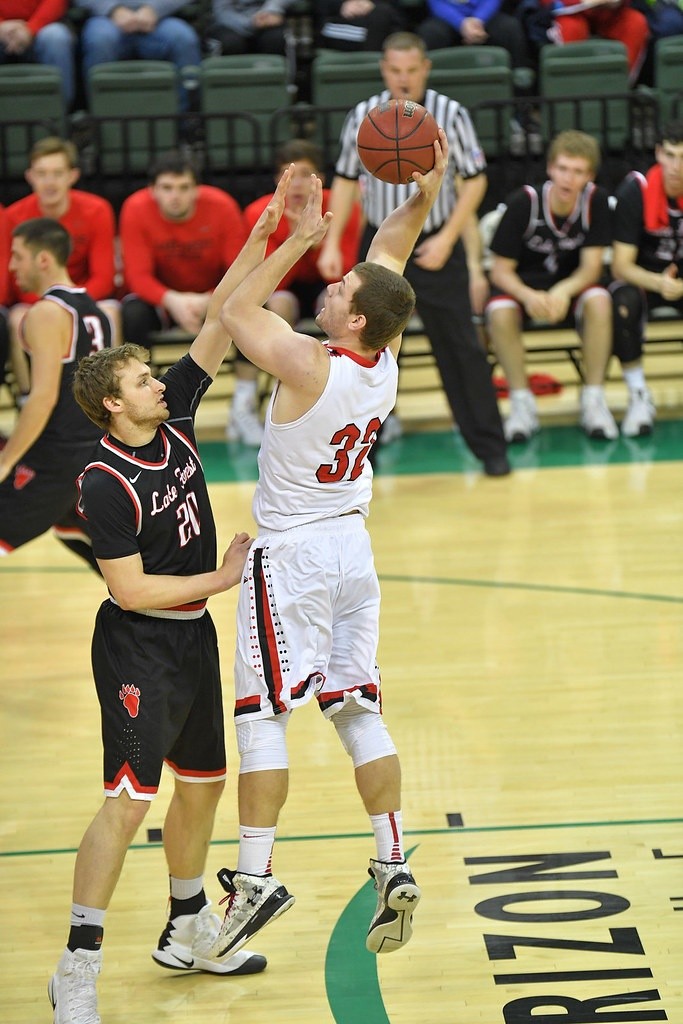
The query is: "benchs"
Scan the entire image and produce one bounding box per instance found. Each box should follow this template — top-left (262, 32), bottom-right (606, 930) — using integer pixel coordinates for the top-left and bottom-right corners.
top-left (0, 305), bottom-right (683, 383)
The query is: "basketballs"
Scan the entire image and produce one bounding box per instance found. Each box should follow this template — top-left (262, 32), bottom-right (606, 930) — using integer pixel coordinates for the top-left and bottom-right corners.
top-left (355, 99), bottom-right (439, 186)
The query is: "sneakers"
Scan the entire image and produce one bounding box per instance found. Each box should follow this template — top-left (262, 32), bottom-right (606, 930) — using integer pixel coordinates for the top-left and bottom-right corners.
top-left (150, 905), bottom-right (267, 976)
top-left (365, 857), bottom-right (421, 954)
top-left (211, 869), bottom-right (297, 960)
top-left (155, 973), bottom-right (268, 1024)
top-left (46, 945), bottom-right (102, 1024)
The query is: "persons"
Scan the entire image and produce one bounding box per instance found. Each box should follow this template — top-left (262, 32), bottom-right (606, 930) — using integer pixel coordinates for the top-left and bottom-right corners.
top-left (0, 219), bottom-right (113, 583)
top-left (0, 137), bottom-right (407, 449)
top-left (481, 128), bottom-right (683, 443)
top-left (201, 125), bottom-right (450, 963)
top-left (0, 0), bottom-right (683, 157)
top-left (317, 28), bottom-right (515, 478)
top-left (43, 161), bottom-right (304, 1023)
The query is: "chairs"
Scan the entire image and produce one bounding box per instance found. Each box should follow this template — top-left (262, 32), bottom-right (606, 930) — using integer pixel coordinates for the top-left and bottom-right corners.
top-left (0, 37), bottom-right (683, 174)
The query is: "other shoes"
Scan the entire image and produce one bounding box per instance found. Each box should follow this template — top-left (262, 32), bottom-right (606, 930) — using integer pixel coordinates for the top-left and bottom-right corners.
top-left (503, 396), bottom-right (541, 442)
top-left (380, 415), bottom-right (404, 445)
top-left (619, 399), bottom-right (660, 436)
top-left (577, 405), bottom-right (619, 440)
top-left (225, 406), bottom-right (266, 447)
top-left (484, 455), bottom-right (511, 477)
